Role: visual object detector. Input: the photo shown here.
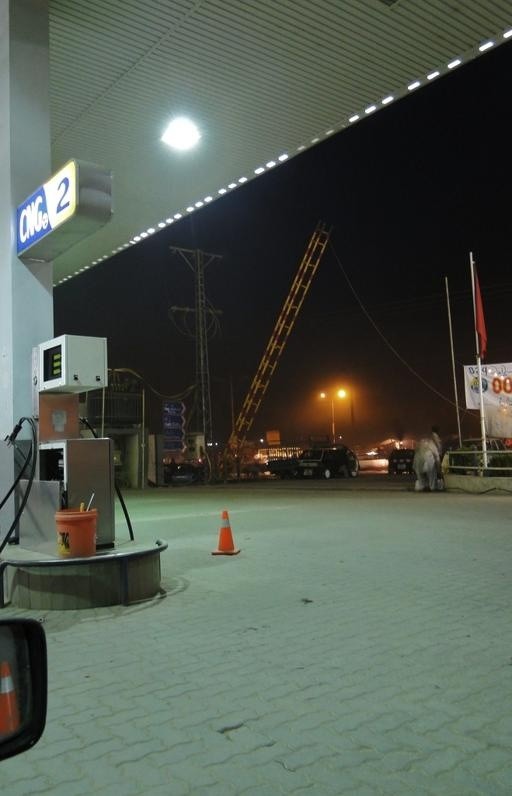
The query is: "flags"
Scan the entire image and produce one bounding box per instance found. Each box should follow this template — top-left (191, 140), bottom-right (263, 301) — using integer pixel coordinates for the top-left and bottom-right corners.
top-left (475, 264), bottom-right (488, 360)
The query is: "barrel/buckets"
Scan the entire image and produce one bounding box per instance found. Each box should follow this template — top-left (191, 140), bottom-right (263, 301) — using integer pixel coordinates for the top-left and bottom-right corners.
top-left (53, 508), bottom-right (99, 560)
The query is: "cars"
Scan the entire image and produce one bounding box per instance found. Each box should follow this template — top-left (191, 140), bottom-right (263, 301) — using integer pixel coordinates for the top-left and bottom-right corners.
top-left (450, 437), bottom-right (508, 467)
top-left (263, 457), bottom-right (298, 476)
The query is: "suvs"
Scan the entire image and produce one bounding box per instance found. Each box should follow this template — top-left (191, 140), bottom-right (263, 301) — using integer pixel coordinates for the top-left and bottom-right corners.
top-left (385, 449), bottom-right (415, 476)
top-left (297, 445), bottom-right (360, 478)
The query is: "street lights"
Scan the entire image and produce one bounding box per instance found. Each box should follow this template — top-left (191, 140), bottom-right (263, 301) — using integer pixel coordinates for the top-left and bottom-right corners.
top-left (319, 388), bottom-right (349, 442)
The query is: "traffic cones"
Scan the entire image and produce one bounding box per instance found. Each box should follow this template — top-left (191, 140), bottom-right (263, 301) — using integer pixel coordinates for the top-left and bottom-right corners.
top-left (0, 657), bottom-right (22, 737)
top-left (210, 508), bottom-right (243, 556)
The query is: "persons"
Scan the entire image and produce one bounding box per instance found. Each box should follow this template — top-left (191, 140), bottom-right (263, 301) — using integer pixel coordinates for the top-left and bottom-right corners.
top-left (411, 437), bottom-right (439, 491)
top-left (430, 426), bottom-right (442, 473)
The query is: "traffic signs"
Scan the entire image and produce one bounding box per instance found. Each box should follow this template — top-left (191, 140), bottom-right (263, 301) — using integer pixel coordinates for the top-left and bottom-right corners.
top-left (163, 427), bottom-right (186, 441)
top-left (163, 440), bottom-right (187, 453)
top-left (162, 401), bottom-right (187, 416)
top-left (162, 414), bottom-right (187, 429)
